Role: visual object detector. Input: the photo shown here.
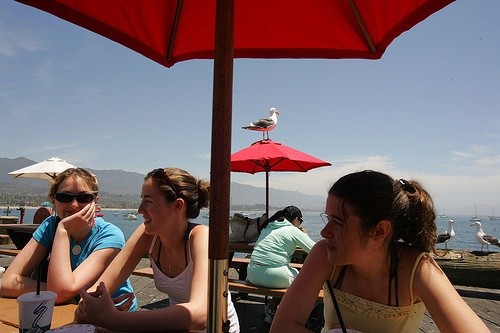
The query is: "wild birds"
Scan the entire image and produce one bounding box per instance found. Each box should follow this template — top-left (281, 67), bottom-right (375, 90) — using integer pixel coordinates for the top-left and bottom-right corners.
top-left (436, 220), bottom-right (456, 249)
top-left (470, 222), bottom-right (500, 252)
top-left (242, 107), bottom-right (280, 141)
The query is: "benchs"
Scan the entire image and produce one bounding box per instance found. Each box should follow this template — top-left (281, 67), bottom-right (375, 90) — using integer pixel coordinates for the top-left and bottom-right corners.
top-left (132, 268), bottom-right (324, 300)
top-left (229, 258), bottom-right (303, 269)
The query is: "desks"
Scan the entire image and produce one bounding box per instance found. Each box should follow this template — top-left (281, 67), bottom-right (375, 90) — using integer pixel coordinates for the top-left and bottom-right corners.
top-left (229, 242), bottom-right (308, 255)
top-left (0, 223), bottom-right (40, 233)
top-left (0, 297), bottom-right (78, 333)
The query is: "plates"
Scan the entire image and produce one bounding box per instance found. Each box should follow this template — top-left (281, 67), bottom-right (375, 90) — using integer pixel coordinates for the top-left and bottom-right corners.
top-left (44, 324), bottom-right (96, 333)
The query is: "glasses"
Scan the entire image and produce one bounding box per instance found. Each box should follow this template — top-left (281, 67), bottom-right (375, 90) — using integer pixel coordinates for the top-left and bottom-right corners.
top-left (294, 215), bottom-right (303, 224)
top-left (53, 192), bottom-right (97, 203)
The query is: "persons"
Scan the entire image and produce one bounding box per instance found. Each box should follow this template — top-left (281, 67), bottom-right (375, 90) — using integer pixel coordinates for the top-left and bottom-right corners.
top-left (0, 168), bottom-right (494, 333)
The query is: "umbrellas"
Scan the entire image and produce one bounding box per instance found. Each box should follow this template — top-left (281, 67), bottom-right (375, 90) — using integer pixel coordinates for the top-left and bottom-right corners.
top-left (230, 139), bottom-right (332, 218)
top-left (7, 156), bottom-right (81, 218)
top-left (13, 0), bottom-right (459, 333)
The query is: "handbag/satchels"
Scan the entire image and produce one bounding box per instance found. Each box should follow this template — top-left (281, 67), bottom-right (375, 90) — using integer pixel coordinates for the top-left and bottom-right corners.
top-left (229, 213), bottom-right (269, 243)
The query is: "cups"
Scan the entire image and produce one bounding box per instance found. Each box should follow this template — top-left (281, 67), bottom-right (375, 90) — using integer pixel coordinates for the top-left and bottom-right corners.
top-left (16, 291), bottom-right (56, 333)
